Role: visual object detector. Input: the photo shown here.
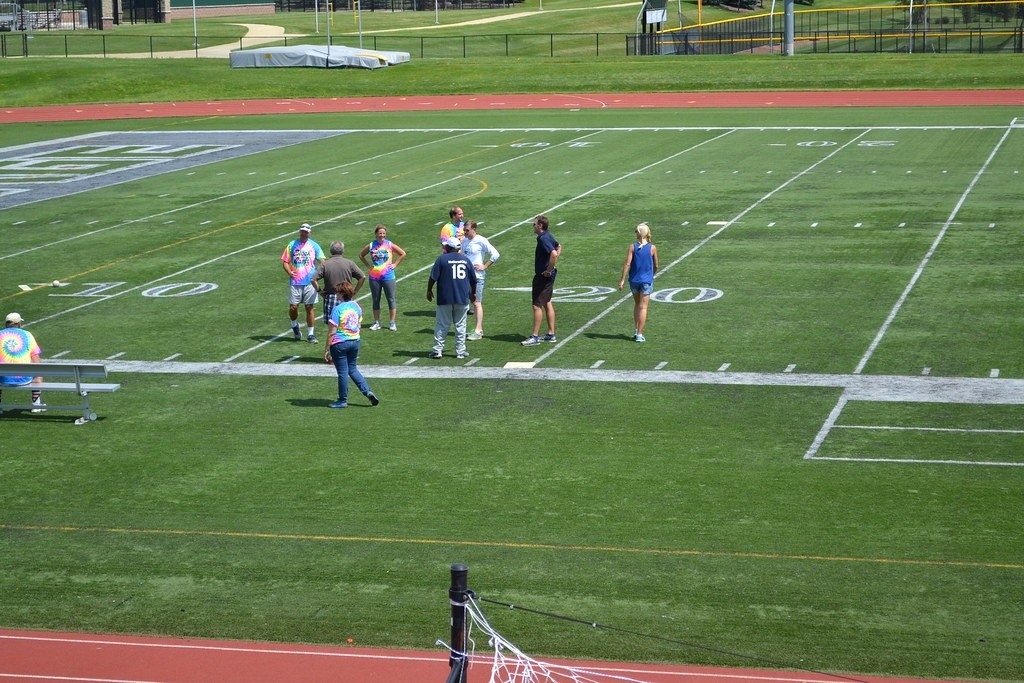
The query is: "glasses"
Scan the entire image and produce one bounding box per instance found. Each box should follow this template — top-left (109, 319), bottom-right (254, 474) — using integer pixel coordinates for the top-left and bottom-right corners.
top-left (532, 223), bottom-right (538, 226)
top-left (301, 226), bottom-right (311, 230)
top-left (635, 230), bottom-right (638, 233)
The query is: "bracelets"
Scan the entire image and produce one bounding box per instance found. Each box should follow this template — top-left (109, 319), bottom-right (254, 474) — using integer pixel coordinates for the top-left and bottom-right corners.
top-left (317, 289), bottom-right (321, 292)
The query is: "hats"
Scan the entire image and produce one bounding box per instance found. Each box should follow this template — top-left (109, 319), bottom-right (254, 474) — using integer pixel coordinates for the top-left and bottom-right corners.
top-left (6, 312), bottom-right (24, 324)
top-left (442, 237), bottom-right (461, 249)
top-left (300, 223), bottom-right (312, 235)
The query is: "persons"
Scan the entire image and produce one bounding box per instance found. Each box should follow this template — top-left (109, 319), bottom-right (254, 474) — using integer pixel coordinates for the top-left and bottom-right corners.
top-left (324, 282), bottom-right (379, 408)
top-left (359, 224), bottom-right (407, 330)
top-left (460, 220), bottom-right (500, 340)
top-left (441, 206), bottom-right (474, 315)
top-left (0, 313), bottom-right (47, 412)
top-left (282, 223), bottom-right (326, 344)
top-left (520, 216), bottom-right (561, 346)
top-left (427, 237), bottom-right (477, 358)
top-left (619, 223), bottom-right (658, 342)
top-left (311, 240), bottom-right (366, 324)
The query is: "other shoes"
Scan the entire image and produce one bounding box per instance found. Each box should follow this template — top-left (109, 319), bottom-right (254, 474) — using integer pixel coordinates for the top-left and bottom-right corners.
top-left (389, 322), bottom-right (396, 331)
top-left (429, 350), bottom-right (442, 358)
top-left (369, 323), bottom-right (382, 330)
top-left (457, 352), bottom-right (469, 358)
top-left (466, 329), bottom-right (484, 340)
top-left (468, 308), bottom-right (475, 314)
top-left (31, 403), bottom-right (47, 413)
top-left (636, 336), bottom-right (645, 343)
top-left (632, 329), bottom-right (638, 338)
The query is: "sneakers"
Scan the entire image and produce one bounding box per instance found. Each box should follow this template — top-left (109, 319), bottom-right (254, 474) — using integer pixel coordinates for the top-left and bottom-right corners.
top-left (367, 392), bottom-right (379, 406)
top-left (328, 398), bottom-right (348, 408)
top-left (307, 335), bottom-right (319, 344)
top-left (292, 323), bottom-right (302, 340)
top-left (521, 336), bottom-right (541, 346)
top-left (538, 334), bottom-right (557, 342)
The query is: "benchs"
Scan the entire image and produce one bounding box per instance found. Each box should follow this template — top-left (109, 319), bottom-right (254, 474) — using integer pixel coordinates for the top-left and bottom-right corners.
top-left (0, 360), bottom-right (121, 421)
